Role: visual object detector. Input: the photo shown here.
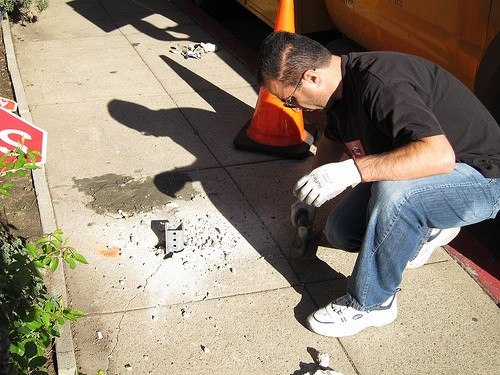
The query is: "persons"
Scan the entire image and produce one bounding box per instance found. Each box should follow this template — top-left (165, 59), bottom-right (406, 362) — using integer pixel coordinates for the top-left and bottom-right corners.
top-left (256, 31), bottom-right (500, 337)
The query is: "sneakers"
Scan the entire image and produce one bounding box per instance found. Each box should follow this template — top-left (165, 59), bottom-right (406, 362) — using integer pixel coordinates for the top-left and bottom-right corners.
top-left (405, 226), bottom-right (460, 269)
top-left (306, 287), bottom-right (401, 337)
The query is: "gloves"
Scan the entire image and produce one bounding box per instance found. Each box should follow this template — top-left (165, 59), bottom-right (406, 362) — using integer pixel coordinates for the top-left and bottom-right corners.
top-left (293, 158), bottom-right (362, 207)
top-left (290, 199), bottom-right (315, 227)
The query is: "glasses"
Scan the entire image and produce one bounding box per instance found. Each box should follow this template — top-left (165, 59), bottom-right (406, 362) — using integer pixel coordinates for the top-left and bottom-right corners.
top-left (284, 77), bottom-right (303, 108)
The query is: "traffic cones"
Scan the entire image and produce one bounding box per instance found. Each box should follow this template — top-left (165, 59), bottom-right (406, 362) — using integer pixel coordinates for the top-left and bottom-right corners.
top-left (230, 0), bottom-right (320, 161)
